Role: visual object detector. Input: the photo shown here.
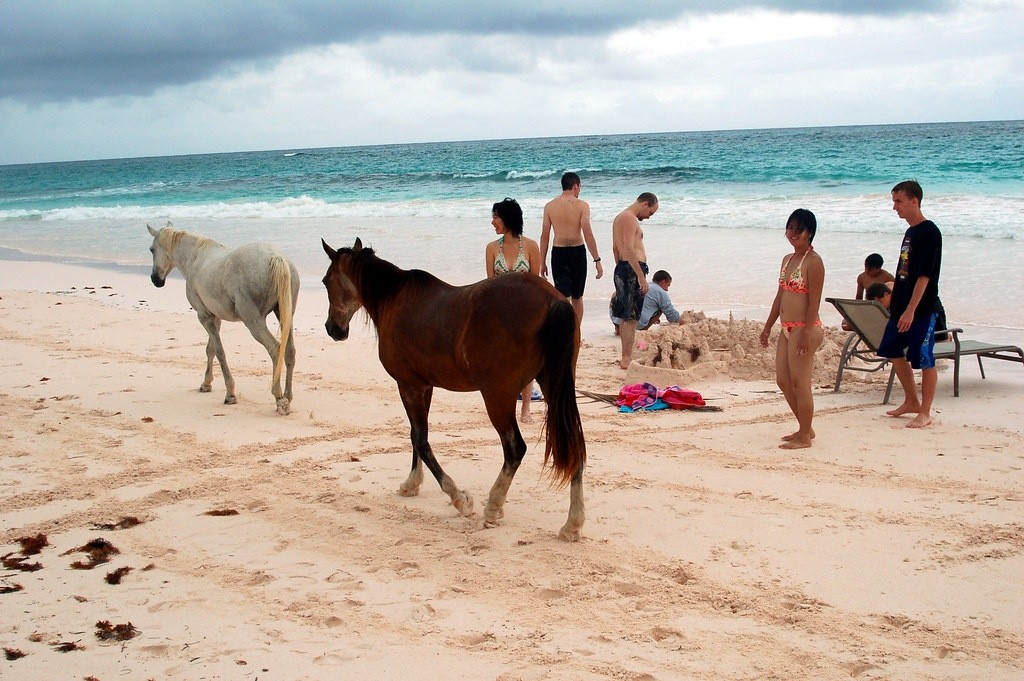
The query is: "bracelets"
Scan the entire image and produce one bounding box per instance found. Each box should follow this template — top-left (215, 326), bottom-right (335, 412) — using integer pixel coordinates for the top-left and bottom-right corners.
top-left (593, 257), bottom-right (601, 262)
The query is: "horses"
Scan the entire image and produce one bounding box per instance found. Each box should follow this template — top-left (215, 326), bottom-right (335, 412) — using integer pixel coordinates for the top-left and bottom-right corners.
top-left (145, 221), bottom-right (300, 416)
top-left (320, 237), bottom-right (586, 541)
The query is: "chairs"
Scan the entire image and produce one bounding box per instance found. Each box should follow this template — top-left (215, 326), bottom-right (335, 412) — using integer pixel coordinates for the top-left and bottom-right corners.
top-left (825, 297), bottom-right (1024, 405)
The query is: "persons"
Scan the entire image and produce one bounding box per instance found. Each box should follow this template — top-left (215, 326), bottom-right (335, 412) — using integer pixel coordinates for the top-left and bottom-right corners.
top-left (876, 180), bottom-right (943, 428)
top-left (609, 270), bottom-right (685, 335)
top-left (540, 172), bottom-right (603, 347)
top-left (486, 198), bottom-right (541, 424)
top-left (865, 282), bottom-right (952, 342)
top-left (613, 192), bottom-right (659, 370)
top-left (759, 208), bottom-right (825, 450)
top-left (842, 253), bottom-right (896, 331)
